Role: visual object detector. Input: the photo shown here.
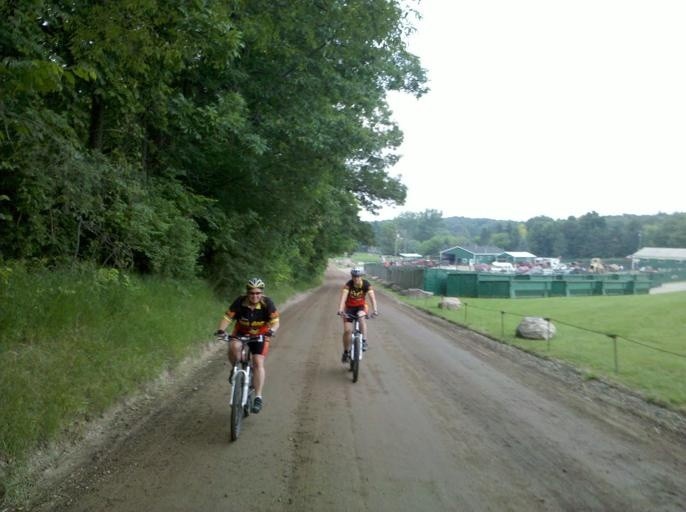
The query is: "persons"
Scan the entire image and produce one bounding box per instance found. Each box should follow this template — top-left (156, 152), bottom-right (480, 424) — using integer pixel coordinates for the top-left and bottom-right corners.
top-left (215, 278), bottom-right (280, 413)
top-left (338, 267), bottom-right (378, 363)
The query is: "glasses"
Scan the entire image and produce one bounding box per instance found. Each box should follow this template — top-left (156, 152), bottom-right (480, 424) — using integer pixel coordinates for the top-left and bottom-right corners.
top-left (249, 292), bottom-right (261, 294)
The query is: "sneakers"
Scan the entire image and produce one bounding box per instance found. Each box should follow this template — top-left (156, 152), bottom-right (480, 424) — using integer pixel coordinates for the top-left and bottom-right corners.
top-left (342, 352), bottom-right (348, 362)
top-left (252, 397), bottom-right (262, 413)
top-left (362, 339), bottom-right (368, 351)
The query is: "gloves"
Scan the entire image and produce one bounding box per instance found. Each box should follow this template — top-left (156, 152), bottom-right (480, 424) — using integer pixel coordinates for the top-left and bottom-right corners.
top-left (337, 312), bottom-right (344, 316)
top-left (213, 330), bottom-right (224, 336)
top-left (264, 330), bottom-right (273, 337)
top-left (371, 312), bottom-right (378, 318)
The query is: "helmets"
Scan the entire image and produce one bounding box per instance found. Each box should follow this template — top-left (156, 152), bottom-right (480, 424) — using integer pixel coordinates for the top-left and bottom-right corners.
top-left (351, 269), bottom-right (361, 276)
top-left (246, 278), bottom-right (265, 289)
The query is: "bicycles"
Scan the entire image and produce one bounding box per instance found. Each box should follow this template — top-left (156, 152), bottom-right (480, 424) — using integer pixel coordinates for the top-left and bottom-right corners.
top-left (217, 333), bottom-right (273, 442)
top-left (337, 310), bottom-right (374, 382)
